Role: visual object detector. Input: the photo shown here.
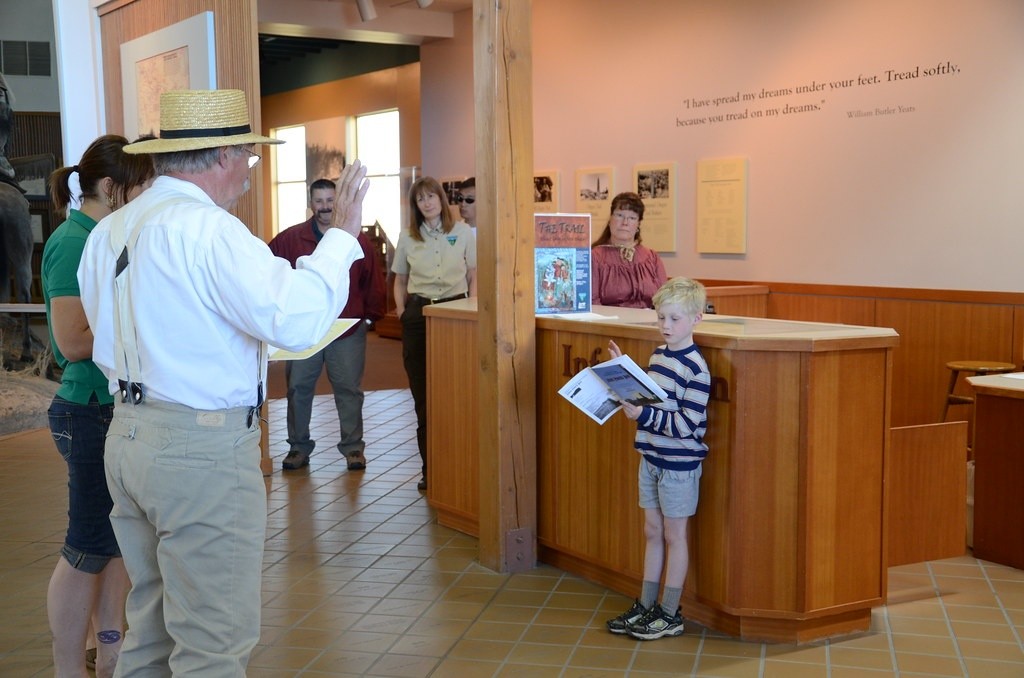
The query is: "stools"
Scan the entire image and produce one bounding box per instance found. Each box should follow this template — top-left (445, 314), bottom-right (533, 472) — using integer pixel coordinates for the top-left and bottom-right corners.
top-left (940, 361), bottom-right (1015, 462)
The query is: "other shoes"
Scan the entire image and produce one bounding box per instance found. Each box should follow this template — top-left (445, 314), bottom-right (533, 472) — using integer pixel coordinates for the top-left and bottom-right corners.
top-left (417, 477), bottom-right (428, 491)
top-left (86, 649), bottom-right (96, 668)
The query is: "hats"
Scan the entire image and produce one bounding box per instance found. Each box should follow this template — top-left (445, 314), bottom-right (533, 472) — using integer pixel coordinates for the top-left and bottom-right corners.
top-left (123, 90), bottom-right (287, 153)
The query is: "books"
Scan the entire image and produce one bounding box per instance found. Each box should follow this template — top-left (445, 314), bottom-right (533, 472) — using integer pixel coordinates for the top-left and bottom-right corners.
top-left (557, 353), bottom-right (668, 425)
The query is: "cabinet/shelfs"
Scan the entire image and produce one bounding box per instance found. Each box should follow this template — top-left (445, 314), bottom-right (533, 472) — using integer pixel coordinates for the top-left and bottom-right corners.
top-left (964, 371), bottom-right (1024, 570)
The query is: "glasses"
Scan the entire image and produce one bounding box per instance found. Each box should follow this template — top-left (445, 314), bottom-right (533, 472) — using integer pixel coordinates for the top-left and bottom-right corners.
top-left (456, 194), bottom-right (474, 204)
top-left (234, 145), bottom-right (261, 169)
top-left (613, 213), bottom-right (639, 224)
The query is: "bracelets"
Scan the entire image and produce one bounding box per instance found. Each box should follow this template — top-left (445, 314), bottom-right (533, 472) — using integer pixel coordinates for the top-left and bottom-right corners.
top-left (366, 319), bottom-right (371, 325)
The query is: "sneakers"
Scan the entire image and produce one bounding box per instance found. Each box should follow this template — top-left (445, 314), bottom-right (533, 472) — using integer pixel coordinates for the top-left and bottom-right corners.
top-left (346, 450), bottom-right (366, 469)
top-left (626, 604), bottom-right (684, 640)
top-left (282, 452), bottom-right (308, 470)
top-left (606, 601), bottom-right (657, 634)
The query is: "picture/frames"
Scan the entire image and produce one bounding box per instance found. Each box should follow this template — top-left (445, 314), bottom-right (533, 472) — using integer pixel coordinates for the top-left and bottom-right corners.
top-left (119, 11), bottom-right (213, 143)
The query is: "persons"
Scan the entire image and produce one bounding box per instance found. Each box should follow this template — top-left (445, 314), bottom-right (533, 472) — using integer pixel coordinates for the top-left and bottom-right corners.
top-left (592, 191), bottom-right (667, 309)
top-left (458, 177), bottom-right (477, 238)
top-left (76, 90), bottom-right (370, 678)
top-left (47, 134), bottom-right (156, 678)
top-left (391, 177), bottom-right (477, 490)
top-left (267, 179), bottom-right (388, 470)
top-left (606, 278), bottom-right (711, 640)
